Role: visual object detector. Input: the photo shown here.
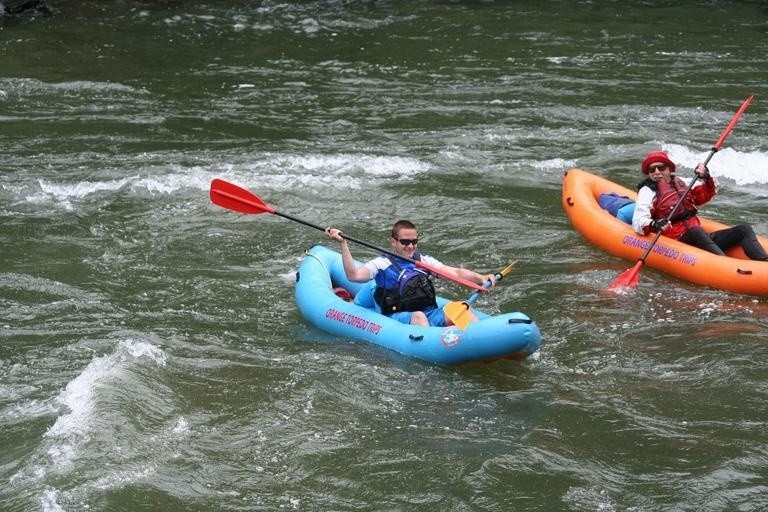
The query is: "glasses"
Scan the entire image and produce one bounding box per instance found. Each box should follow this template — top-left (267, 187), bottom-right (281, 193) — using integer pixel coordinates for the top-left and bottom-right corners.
top-left (394, 237), bottom-right (420, 245)
top-left (646, 164), bottom-right (667, 173)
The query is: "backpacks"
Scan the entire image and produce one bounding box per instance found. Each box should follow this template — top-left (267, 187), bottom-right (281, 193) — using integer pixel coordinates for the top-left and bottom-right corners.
top-left (382, 253), bottom-right (435, 310)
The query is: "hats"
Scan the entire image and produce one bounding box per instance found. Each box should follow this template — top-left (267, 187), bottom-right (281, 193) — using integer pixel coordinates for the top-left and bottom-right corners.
top-left (642, 152), bottom-right (675, 174)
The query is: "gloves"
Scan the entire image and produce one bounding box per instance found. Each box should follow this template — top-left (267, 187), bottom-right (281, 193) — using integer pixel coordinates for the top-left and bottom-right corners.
top-left (694, 163), bottom-right (710, 179)
top-left (650, 219), bottom-right (668, 232)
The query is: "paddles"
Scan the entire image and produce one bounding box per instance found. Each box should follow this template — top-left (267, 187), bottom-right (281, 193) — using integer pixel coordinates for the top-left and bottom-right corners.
top-left (209, 178), bottom-right (492, 294)
top-left (443, 258), bottom-right (515, 328)
top-left (612, 91), bottom-right (757, 290)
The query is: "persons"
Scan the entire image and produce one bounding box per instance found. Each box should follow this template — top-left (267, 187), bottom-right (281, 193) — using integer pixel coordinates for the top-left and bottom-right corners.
top-left (632, 149), bottom-right (768, 263)
top-left (324, 217), bottom-right (498, 328)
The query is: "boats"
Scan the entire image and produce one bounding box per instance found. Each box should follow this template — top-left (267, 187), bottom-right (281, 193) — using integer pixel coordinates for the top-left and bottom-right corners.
top-left (562, 168), bottom-right (768, 296)
top-left (295, 245), bottom-right (542, 366)
